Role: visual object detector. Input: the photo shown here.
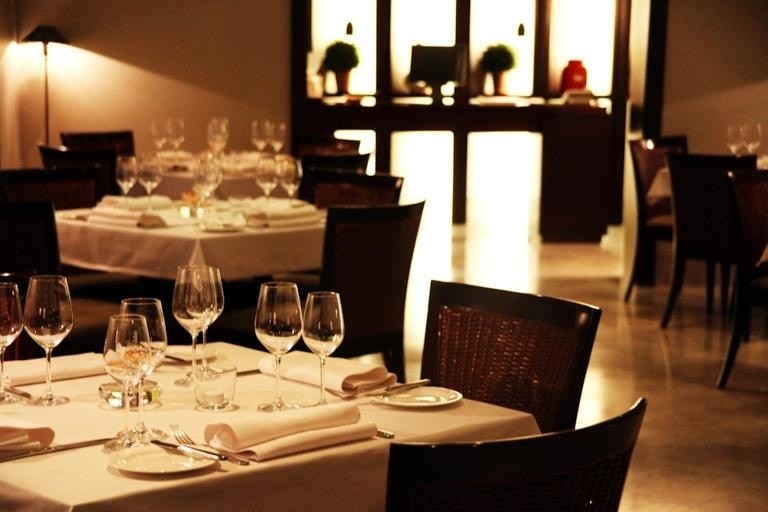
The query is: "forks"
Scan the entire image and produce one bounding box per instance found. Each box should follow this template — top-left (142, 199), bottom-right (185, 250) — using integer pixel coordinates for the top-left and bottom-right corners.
top-left (169, 423), bottom-right (250, 467)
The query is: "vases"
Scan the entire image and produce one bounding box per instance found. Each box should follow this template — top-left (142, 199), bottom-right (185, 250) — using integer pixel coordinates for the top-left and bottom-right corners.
top-left (560, 60), bottom-right (587, 96)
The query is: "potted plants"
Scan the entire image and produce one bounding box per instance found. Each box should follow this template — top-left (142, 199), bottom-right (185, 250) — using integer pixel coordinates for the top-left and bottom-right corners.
top-left (325, 42), bottom-right (359, 97)
top-left (479, 45), bottom-right (514, 98)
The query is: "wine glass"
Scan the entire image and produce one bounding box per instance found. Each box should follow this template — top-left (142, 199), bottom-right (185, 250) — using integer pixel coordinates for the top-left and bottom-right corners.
top-left (118, 298), bottom-right (169, 439)
top-left (118, 115), bottom-right (305, 231)
top-left (20, 273), bottom-right (74, 406)
top-left (103, 314), bottom-right (153, 450)
top-left (301, 291), bottom-right (345, 408)
top-left (184, 264), bottom-right (224, 379)
top-left (254, 281), bottom-right (303, 411)
top-left (725, 124), bottom-right (767, 171)
top-left (1, 272), bottom-right (33, 405)
top-left (171, 265), bottom-right (217, 386)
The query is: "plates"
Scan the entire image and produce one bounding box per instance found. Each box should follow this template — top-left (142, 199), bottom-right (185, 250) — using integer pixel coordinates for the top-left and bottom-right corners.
top-left (368, 383), bottom-right (461, 410)
top-left (108, 443), bottom-right (221, 475)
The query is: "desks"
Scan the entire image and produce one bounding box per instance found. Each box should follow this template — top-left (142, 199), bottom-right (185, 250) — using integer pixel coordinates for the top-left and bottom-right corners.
top-left (298, 98), bottom-right (623, 242)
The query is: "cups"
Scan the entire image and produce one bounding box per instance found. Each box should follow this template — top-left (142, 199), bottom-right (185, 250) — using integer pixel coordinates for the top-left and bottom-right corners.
top-left (189, 361), bottom-right (240, 411)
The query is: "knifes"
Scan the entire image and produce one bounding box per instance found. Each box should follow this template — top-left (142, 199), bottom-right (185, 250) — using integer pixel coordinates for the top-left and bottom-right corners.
top-left (355, 379), bottom-right (430, 396)
top-left (0, 436), bottom-right (117, 464)
top-left (152, 439), bottom-right (225, 461)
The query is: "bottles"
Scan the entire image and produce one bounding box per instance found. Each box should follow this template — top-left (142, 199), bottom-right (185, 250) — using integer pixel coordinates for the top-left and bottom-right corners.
top-left (563, 60), bottom-right (586, 92)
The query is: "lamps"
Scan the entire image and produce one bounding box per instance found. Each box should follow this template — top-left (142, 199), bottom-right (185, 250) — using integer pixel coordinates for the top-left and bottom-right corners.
top-left (23, 26), bottom-right (71, 174)
top-left (413, 46), bottom-right (468, 105)
